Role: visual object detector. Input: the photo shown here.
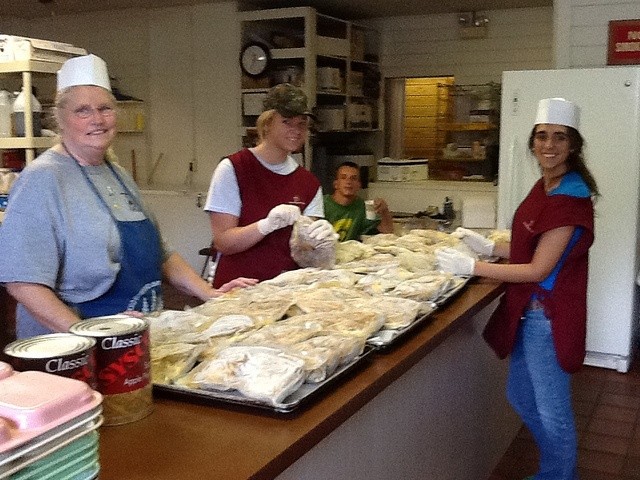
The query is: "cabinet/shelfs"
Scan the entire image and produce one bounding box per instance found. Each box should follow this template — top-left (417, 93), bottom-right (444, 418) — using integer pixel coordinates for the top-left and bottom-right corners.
top-left (0, 58), bottom-right (74, 224)
top-left (241, 6), bottom-right (386, 181)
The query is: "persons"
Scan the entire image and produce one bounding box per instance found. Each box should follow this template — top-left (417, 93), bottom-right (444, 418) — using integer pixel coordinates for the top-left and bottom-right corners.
top-left (203, 83), bottom-right (341, 289)
top-left (434, 98), bottom-right (601, 479)
top-left (0, 54), bottom-right (260, 338)
top-left (323, 162), bottom-right (392, 242)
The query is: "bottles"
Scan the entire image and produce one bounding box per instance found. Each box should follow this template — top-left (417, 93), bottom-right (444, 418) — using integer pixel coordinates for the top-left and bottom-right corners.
top-left (0, 88), bottom-right (9, 138)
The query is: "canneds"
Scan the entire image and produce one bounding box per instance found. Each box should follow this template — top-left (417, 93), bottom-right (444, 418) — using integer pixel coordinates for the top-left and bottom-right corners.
top-left (67, 315), bottom-right (154, 426)
top-left (4, 333), bottom-right (99, 394)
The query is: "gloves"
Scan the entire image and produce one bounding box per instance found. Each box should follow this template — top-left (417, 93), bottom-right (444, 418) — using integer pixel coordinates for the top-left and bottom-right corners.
top-left (257, 203), bottom-right (300, 237)
top-left (307, 219), bottom-right (340, 241)
top-left (434, 248), bottom-right (475, 276)
top-left (462, 228), bottom-right (494, 257)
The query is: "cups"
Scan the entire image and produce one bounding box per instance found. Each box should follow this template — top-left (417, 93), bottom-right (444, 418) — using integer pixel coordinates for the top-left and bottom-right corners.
top-left (365, 200), bottom-right (375, 219)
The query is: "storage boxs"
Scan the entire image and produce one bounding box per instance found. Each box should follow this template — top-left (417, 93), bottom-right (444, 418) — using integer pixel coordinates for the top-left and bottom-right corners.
top-left (0, 35), bottom-right (87, 62)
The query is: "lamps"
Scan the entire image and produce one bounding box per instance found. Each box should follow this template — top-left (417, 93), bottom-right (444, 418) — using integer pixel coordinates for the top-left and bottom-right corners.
top-left (457, 14), bottom-right (490, 40)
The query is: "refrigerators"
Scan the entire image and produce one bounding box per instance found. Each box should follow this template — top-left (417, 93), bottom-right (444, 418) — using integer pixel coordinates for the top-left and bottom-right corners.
top-left (497, 67), bottom-right (640, 372)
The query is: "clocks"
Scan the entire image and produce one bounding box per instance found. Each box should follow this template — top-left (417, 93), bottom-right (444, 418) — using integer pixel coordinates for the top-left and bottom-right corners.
top-left (240, 42), bottom-right (271, 78)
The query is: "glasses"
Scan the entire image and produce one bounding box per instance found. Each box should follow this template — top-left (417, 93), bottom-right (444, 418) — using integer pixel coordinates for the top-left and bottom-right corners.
top-left (59, 104), bottom-right (113, 120)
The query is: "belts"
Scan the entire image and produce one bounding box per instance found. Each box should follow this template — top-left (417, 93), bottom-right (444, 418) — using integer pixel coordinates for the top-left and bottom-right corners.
top-left (526, 300), bottom-right (546, 309)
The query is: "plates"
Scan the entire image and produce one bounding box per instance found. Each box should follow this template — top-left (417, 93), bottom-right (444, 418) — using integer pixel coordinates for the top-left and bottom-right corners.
top-left (187, 254), bottom-right (502, 345)
top-left (0, 360), bottom-right (104, 480)
top-left (151, 346), bottom-right (372, 412)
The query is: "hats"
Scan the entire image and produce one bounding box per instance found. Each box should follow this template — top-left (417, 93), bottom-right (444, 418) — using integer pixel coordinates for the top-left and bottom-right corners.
top-left (263, 83), bottom-right (321, 125)
top-left (534, 98), bottom-right (580, 130)
top-left (56, 53), bottom-right (111, 91)
